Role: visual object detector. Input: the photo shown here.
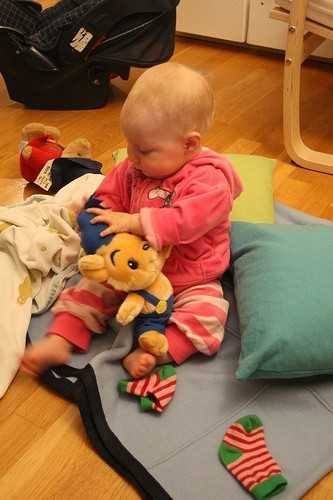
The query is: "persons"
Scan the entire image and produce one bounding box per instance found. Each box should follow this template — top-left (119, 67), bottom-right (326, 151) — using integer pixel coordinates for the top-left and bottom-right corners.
top-left (21, 61), bottom-right (244, 378)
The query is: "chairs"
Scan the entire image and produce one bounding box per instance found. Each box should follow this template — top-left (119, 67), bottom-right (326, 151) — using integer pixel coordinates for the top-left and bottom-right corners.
top-left (269, 0), bottom-right (333, 177)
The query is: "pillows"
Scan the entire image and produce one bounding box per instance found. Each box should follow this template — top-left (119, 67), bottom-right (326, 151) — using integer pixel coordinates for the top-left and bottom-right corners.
top-left (230, 220), bottom-right (332, 381)
top-left (112, 148), bottom-right (276, 224)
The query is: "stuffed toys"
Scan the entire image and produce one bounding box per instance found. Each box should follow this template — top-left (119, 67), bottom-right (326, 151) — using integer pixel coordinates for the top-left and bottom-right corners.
top-left (17, 121), bottom-right (103, 200)
top-left (77, 207), bottom-right (175, 356)
